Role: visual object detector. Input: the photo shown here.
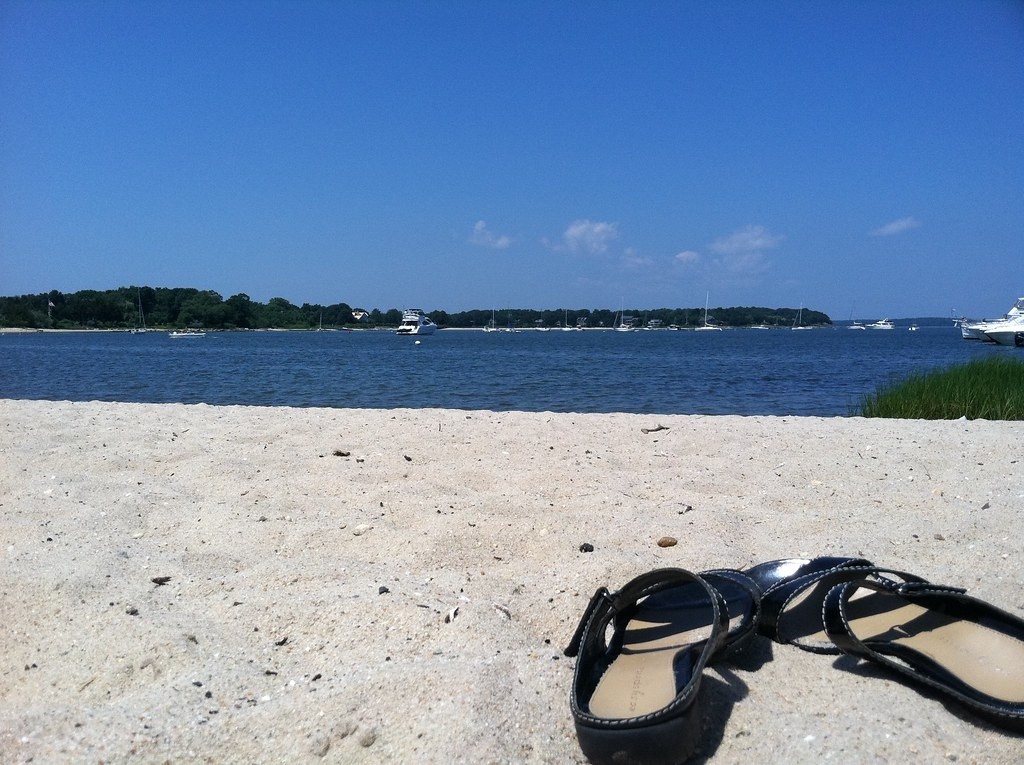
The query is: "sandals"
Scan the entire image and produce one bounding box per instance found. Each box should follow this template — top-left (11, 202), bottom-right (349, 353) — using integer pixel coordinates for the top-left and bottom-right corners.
top-left (567, 567), bottom-right (761, 765)
top-left (738, 556), bottom-right (1023, 727)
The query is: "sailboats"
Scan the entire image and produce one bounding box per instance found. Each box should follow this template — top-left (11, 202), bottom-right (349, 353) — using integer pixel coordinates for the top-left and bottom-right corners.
top-left (612, 289), bottom-right (723, 331)
top-left (791, 302), bottom-right (814, 330)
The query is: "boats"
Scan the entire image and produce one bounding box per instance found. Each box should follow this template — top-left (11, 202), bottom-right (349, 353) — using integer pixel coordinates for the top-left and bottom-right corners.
top-left (960, 296), bottom-right (1024, 348)
top-left (847, 316), bottom-right (920, 330)
top-left (169, 329), bottom-right (206, 339)
top-left (482, 324), bottom-right (585, 332)
top-left (750, 325), bottom-right (771, 329)
top-left (395, 309), bottom-right (438, 334)
